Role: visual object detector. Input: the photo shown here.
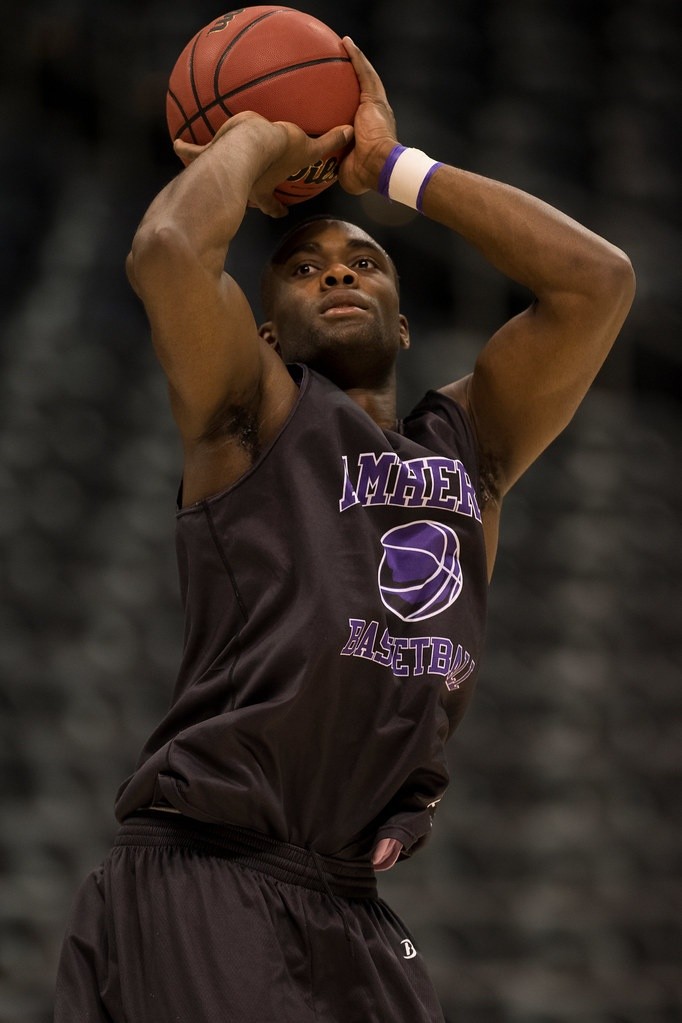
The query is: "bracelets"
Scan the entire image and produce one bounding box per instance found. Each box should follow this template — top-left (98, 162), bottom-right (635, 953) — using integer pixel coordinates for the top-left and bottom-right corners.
top-left (378, 145), bottom-right (443, 213)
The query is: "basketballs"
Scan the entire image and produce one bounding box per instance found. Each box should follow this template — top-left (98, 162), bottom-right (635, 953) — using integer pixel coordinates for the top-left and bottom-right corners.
top-left (166, 5), bottom-right (363, 208)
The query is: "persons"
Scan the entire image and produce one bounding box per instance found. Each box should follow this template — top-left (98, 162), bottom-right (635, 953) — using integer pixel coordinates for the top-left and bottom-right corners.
top-left (59, 39), bottom-right (637, 1023)
